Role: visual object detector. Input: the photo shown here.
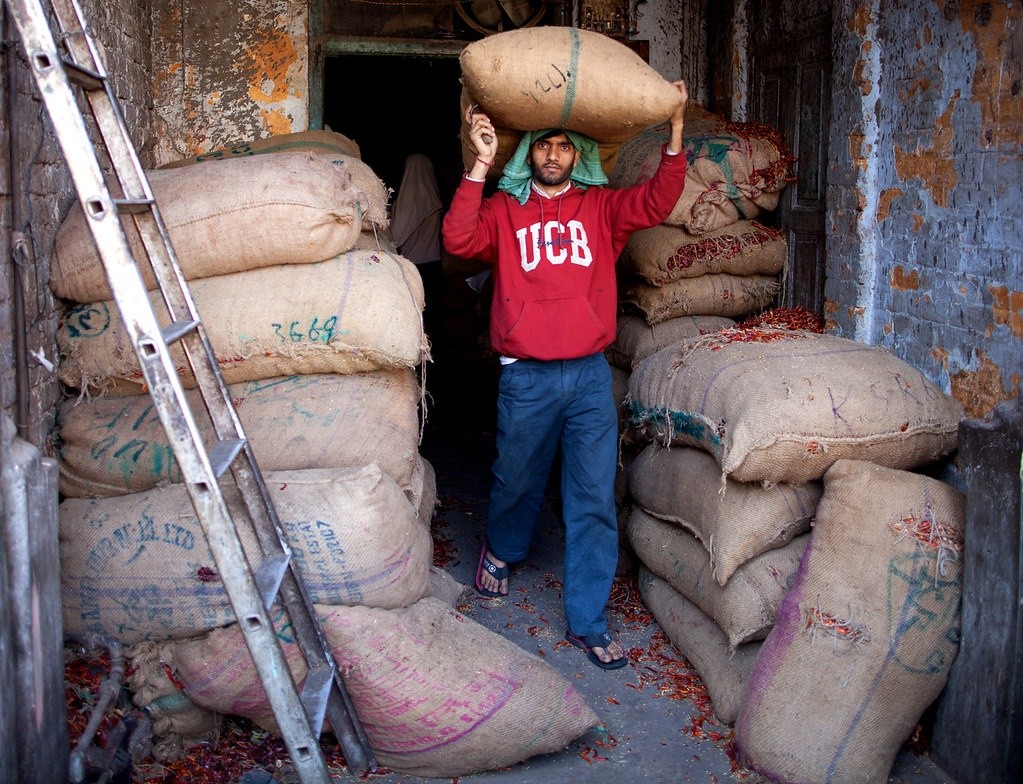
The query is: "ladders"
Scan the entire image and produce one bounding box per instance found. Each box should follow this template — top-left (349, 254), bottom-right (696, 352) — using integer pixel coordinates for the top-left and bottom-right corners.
top-left (10, 0), bottom-right (381, 784)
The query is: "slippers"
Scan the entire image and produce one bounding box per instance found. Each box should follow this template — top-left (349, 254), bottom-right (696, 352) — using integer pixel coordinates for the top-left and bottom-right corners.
top-left (564, 629), bottom-right (627, 669)
top-left (476, 536), bottom-right (509, 596)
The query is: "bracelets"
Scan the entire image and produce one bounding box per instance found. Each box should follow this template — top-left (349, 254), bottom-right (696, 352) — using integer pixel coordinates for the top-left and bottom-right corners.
top-left (475, 157), bottom-right (495, 168)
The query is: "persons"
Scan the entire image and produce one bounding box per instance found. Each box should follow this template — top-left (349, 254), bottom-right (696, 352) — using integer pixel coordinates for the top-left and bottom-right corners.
top-left (441, 79), bottom-right (690, 670)
top-left (389, 154), bottom-right (442, 274)
